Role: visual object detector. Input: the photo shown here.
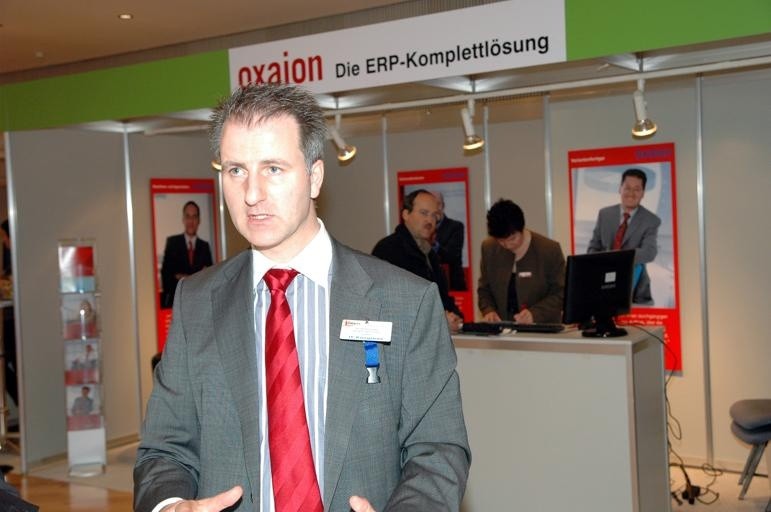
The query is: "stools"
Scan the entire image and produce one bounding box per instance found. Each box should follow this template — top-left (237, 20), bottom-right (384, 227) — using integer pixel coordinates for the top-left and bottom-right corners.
top-left (728, 399), bottom-right (770, 501)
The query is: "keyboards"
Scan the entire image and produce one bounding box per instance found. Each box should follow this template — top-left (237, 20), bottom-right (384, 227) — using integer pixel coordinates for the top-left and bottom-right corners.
top-left (458, 322), bottom-right (565, 335)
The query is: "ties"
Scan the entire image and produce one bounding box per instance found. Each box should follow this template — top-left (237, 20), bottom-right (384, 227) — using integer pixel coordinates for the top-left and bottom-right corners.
top-left (613, 213), bottom-right (630, 252)
top-left (262, 269), bottom-right (327, 512)
top-left (188, 240), bottom-right (194, 267)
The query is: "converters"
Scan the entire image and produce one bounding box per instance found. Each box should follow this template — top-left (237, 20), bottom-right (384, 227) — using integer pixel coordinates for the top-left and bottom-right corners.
top-left (682, 486), bottom-right (700, 501)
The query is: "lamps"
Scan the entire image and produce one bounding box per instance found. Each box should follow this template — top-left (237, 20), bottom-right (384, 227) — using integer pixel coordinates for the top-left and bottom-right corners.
top-left (630, 78), bottom-right (657, 139)
top-left (328, 114), bottom-right (356, 161)
top-left (460, 98), bottom-right (484, 150)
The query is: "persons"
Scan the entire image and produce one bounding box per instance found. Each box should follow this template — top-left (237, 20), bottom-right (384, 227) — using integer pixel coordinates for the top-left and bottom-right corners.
top-left (73, 302), bottom-right (94, 331)
top-left (131, 86), bottom-right (471, 512)
top-left (371, 188), bottom-right (464, 332)
top-left (586, 169), bottom-right (660, 305)
top-left (74, 343), bottom-right (96, 371)
top-left (160, 201), bottom-right (212, 309)
top-left (476, 199), bottom-right (566, 323)
top-left (428, 192), bottom-right (464, 291)
top-left (71, 387), bottom-right (92, 419)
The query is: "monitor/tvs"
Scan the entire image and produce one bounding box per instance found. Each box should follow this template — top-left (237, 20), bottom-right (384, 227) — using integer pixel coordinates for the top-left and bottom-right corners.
top-left (562, 249), bottom-right (637, 339)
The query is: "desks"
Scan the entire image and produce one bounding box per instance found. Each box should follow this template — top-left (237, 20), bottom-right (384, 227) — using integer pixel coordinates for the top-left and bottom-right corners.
top-left (450, 326), bottom-right (671, 511)
